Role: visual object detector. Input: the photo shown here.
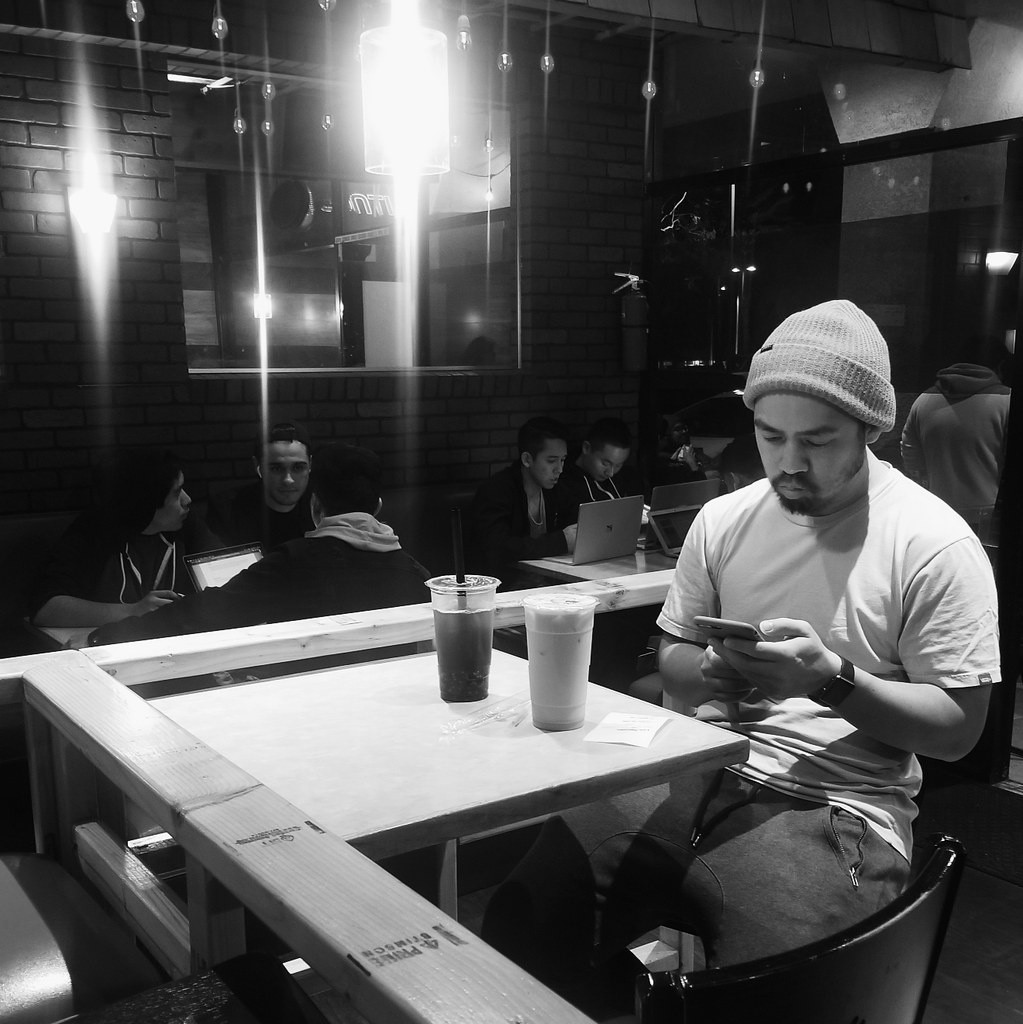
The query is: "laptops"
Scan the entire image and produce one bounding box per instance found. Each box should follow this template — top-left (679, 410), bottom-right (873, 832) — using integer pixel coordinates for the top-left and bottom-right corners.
top-left (648, 504), bottom-right (703, 558)
top-left (637, 478), bottom-right (723, 550)
top-left (184, 541), bottom-right (266, 592)
top-left (542, 495), bottom-right (646, 566)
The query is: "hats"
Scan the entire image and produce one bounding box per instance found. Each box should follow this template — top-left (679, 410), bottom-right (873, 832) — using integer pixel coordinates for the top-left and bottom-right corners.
top-left (743, 300), bottom-right (896, 432)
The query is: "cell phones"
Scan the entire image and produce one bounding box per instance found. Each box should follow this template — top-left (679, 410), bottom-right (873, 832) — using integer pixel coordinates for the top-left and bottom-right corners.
top-left (692, 616), bottom-right (766, 645)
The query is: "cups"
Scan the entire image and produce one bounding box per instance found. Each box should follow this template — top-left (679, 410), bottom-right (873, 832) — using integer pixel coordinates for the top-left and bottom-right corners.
top-left (523, 593), bottom-right (601, 731)
top-left (425, 574), bottom-right (501, 703)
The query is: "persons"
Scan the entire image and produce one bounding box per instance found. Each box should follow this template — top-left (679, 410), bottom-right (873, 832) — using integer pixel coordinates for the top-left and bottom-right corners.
top-left (206, 424), bottom-right (316, 550)
top-left (87, 443), bottom-right (433, 647)
top-left (469, 414), bottom-right (707, 673)
top-left (900, 332), bottom-right (1012, 543)
top-left (478, 299), bottom-right (1001, 1019)
top-left (27, 443), bottom-right (228, 629)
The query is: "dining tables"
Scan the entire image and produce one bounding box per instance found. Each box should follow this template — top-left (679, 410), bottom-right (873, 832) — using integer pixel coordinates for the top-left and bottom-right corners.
top-left (0, 539), bottom-right (750, 1024)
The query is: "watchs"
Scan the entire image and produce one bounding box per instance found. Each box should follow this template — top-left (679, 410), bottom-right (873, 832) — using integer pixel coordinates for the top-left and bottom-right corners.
top-left (807, 655), bottom-right (855, 708)
